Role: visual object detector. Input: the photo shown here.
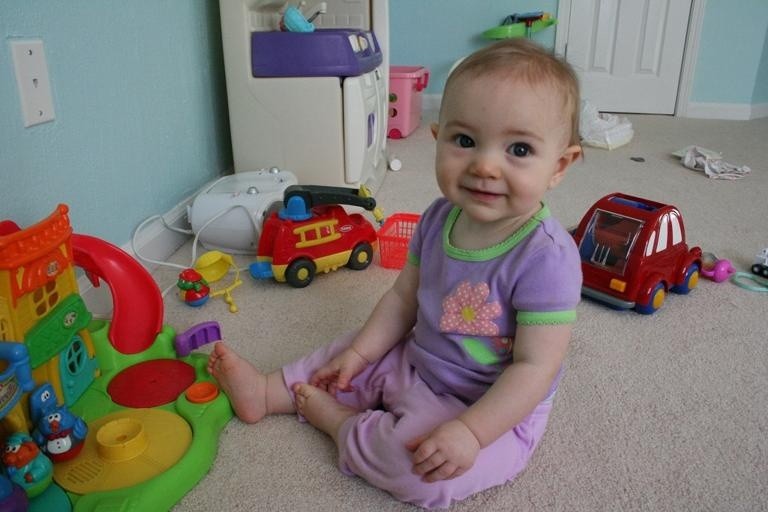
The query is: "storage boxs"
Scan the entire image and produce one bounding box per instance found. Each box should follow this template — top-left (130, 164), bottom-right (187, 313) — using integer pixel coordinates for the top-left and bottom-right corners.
top-left (386, 64), bottom-right (429, 140)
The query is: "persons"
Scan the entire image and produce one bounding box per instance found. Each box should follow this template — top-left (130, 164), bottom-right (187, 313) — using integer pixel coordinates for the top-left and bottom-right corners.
top-left (204, 38), bottom-right (587, 509)
